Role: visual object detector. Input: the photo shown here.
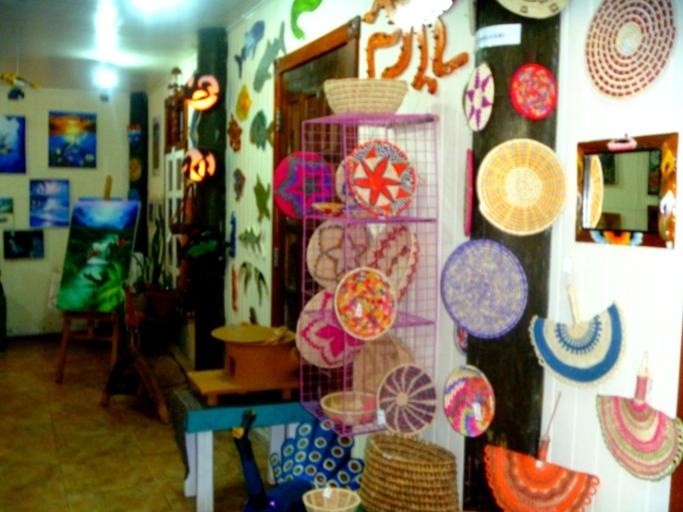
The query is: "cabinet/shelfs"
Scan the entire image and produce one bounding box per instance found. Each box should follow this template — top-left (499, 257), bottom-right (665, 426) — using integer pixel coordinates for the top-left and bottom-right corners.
top-left (298, 113), bottom-right (436, 440)
top-left (160, 95), bottom-right (190, 288)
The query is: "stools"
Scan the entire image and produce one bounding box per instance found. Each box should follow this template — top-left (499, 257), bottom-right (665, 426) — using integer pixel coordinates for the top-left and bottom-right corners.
top-left (170, 392), bottom-right (323, 512)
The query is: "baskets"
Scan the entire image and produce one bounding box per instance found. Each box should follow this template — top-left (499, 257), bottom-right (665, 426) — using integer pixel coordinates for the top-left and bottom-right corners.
top-left (359, 434), bottom-right (460, 512)
top-left (324, 77), bottom-right (408, 114)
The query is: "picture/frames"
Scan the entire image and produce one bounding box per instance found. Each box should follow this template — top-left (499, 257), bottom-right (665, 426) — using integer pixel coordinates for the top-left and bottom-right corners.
top-left (47, 110), bottom-right (97, 170)
top-left (3, 229), bottom-right (44, 259)
top-left (575, 131), bottom-right (676, 249)
top-left (30, 179), bottom-right (71, 228)
top-left (0, 115), bottom-right (25, 176)
top-left (1, 197), bottom-right (15, 230)
top-left (54, 197), bottom-right (142, 313)
